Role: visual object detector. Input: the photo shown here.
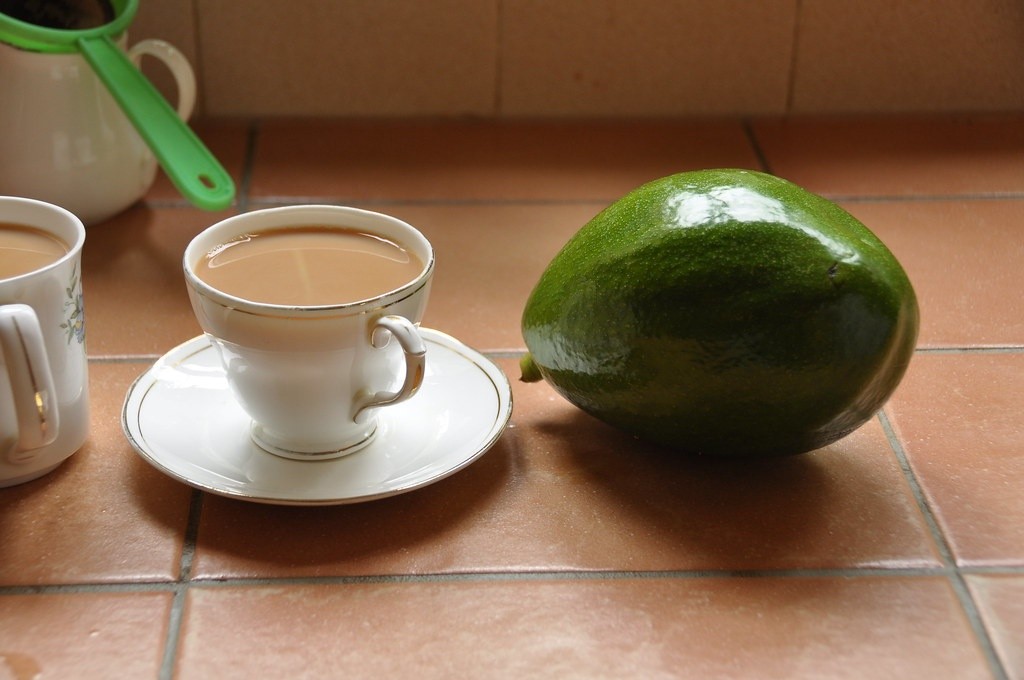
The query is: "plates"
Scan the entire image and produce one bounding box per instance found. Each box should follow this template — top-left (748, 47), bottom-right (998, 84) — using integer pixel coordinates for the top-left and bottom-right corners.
top-left (121, 325), bottom-right (513, 507)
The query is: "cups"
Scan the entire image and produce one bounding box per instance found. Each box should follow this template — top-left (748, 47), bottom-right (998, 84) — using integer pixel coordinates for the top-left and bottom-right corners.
top-left (0, 30), bottom-right (198, 231)
top-left (0, 197), bottom-right (88, 488)
top-left (183, 205), bottom-right (437, 460)
